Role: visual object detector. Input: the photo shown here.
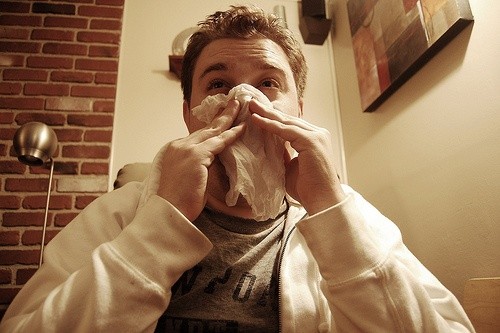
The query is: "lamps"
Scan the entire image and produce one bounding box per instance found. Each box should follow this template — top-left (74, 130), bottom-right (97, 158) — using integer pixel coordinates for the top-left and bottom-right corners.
top-left (13, 122), bottom-right (58, 268)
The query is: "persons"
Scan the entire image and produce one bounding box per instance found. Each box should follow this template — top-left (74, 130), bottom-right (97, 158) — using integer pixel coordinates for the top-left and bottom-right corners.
top-left (1, 4), bottom-right (477, 333)
